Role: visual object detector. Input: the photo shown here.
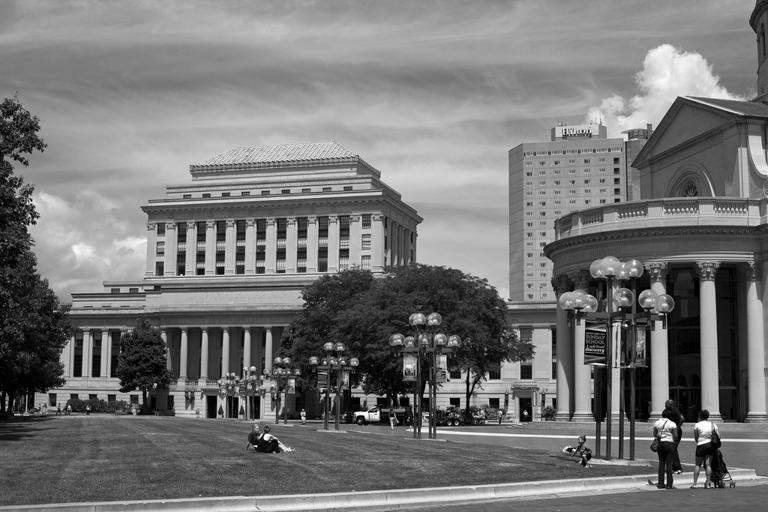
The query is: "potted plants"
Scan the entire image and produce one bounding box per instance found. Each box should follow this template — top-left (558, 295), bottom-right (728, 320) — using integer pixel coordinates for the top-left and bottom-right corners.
top-left (541, 404), bottom-right (557, 420)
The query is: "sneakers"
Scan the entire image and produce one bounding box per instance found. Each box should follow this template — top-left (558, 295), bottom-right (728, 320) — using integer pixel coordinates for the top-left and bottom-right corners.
top-left (657, 483), bottom-right (678, 489)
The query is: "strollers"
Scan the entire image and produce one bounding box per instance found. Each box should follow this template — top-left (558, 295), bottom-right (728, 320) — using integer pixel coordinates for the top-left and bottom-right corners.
top-left (705, 448), bottom-right (735, 489)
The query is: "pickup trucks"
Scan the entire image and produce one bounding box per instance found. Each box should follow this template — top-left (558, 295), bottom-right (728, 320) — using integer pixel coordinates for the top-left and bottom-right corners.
top-left (354, 405), bottom-right (406, 426)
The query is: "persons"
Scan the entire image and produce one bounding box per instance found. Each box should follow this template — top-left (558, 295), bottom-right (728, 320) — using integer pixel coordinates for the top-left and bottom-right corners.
top-left (387, 408), bottom-right (530, 430)
top-left (653, 400), bottom-right (721, 489)
top-left (300, 409), bottom-right (306, 425)
top-left (245, 423), bottom-right (296, 453)
top-left (39, 403), bottom-right (92, 415)
top-left (572, 435), bottom-right (592, 469)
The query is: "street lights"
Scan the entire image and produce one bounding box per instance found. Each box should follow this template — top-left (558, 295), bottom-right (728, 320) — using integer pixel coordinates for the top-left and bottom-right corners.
top-left (558, 254), bottom-right (677, 463)
top-left (309, 342), bottom-right (360, 431)
top-left (388, 311), bottom-right (463, 440)
top-left (216, 365), bottom-right (264, 421)
top-left (263, 357), bottom-right (302, 424)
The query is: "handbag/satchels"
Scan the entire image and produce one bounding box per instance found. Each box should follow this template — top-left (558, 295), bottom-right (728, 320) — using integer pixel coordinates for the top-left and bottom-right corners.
top-left (650, 438), bottom-right (659, 452)
top-left (712, 422), bottom-right (721, 448)
